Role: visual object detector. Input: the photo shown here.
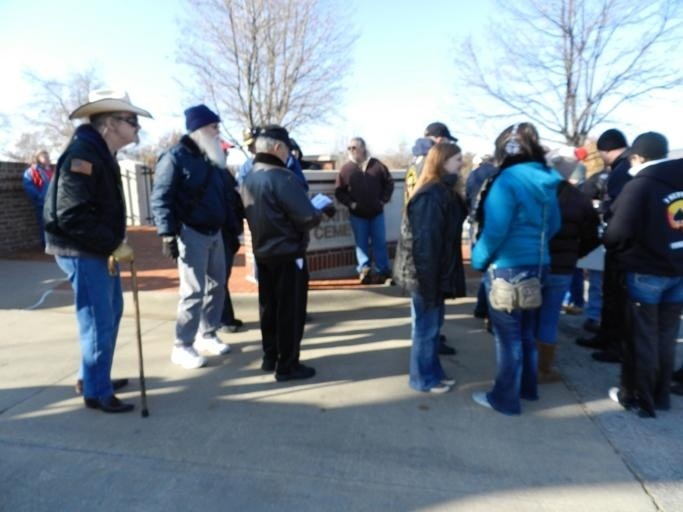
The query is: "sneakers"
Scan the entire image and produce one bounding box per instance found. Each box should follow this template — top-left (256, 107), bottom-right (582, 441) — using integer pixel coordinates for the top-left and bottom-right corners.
top-left (261, 356), bottom-right (275, 371)
top-left (170, 346), bottom-right (207, 368)
top-left (576, 317), bottom-right (683, 403)
top-left (359, 267), bottom-right (371, 283)
top-left (222, 319), bottom-right (243, 333)
top-left (430, 334), bottom-right (492, 408)
top-left (275, 364), bottom-right (316, 381)
top-left (194, 333), bottom-right (231, 354)
top-left (378, 273), bottom-right (395, 286)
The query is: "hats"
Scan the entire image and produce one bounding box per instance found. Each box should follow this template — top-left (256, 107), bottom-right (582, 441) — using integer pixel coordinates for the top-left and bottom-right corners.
top-left (220, 126), bottom-right (293, 150)
top-left (411, 137), bottom-right (436, 156)
top-left (424, 121), bottom-right (457, 141)
top-left (618, 131), bottom-right (669, 159)
top-left (184, 103), bottom-right (222, 132)
top-left (596, 128), bottom-right (626, 150)
top-left (68, 88), bottom-right (153, 120)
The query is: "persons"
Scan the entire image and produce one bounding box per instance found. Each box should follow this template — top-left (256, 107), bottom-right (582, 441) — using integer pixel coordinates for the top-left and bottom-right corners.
top-left (23, 151), bottom-right (53, 252)
top-left (242, 125), bottom-right (334, 382)
top-left (217, 138), bottom-right (246, 333)
top-left (290, 136), bottom-right (320, 170)
top-left (394, 123), bottom-right (469, 393)
top-left (463, 123), bottom-right (683, 420)
top-left (42, 89), bottom-right (154, 411)
top-left (335, 137), bottom-right (394, 284)
top-left (150, 104), bottom-right (232, 369)
top-left (237, 126), bottom-right (309, 281)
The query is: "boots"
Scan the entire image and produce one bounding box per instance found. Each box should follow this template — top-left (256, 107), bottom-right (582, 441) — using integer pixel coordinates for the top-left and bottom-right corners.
top-left (537, 341), bottom-right (563, 384)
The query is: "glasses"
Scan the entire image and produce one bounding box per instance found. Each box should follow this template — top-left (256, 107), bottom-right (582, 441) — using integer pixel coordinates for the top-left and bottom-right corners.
top-left (114, 114), bottom-right (139, 128)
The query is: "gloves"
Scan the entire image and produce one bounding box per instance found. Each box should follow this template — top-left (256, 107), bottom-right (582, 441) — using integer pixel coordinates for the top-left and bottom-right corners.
top-left (113, 243), bottom-right (134, 265)
top-left (161, 238), bottom-right (179, 259)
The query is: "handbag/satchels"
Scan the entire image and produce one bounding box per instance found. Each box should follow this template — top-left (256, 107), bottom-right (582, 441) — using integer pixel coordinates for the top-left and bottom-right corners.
top-left (488, 275), bottom-right (545, 312)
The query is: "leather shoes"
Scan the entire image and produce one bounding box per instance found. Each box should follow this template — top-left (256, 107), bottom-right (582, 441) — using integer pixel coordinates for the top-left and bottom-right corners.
top-left (75, 376), bottom-right (129, 393)
top-left (84, 393), bottom-right (134, 412)
